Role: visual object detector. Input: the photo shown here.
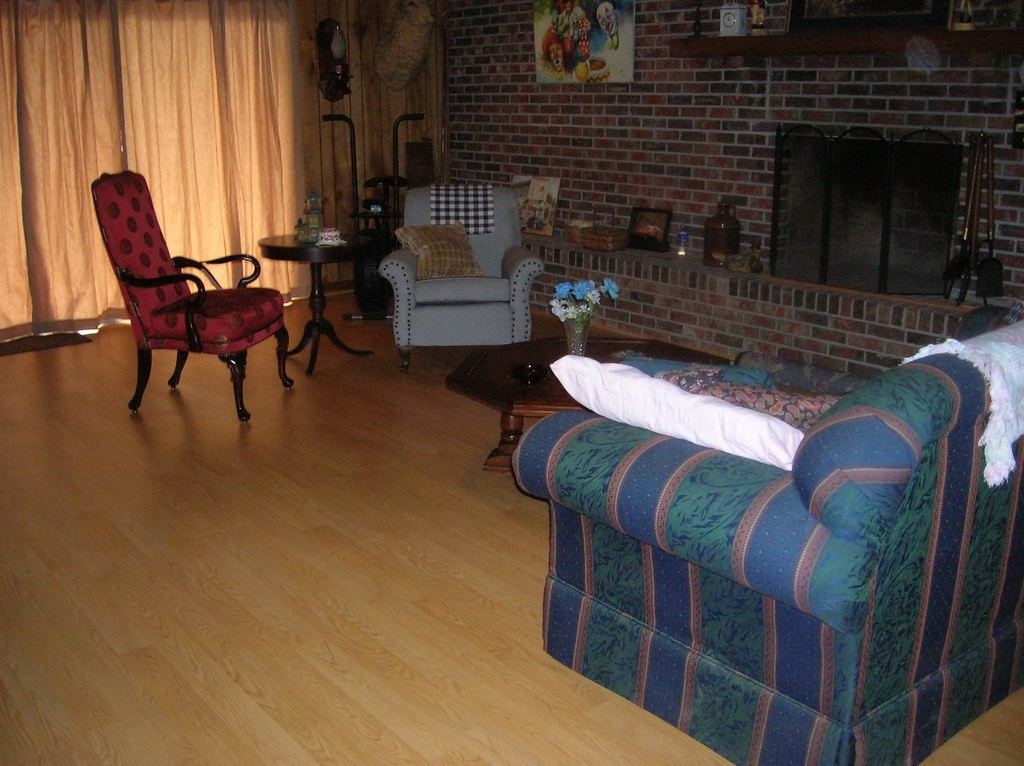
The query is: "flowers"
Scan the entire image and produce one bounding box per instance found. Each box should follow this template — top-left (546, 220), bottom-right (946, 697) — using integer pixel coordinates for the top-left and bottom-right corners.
top-left (550, 278), bottom-right (619, 351)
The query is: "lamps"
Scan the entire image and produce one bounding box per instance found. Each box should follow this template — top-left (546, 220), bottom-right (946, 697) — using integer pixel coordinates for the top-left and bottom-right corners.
top-left (303, 184), bottom-right (325, 241)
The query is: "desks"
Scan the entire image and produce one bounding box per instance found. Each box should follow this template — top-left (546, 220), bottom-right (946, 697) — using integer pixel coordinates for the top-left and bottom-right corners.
top-left (257, 230), bottom-right (382, 376)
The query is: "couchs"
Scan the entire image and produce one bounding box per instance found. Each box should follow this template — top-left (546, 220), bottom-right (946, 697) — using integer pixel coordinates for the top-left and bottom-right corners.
top-left (512, 312), bottom-right (1024, 766)
top-left (378, 184), bottom-right (544, 371)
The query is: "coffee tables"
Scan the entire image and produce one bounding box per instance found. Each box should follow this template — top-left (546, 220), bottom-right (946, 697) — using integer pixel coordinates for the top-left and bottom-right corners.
top-left (444, 337), bottom-right (693, 474)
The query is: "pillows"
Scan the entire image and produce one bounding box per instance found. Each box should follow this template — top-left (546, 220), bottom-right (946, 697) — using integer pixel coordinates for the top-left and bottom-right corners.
top-left (395, 222), bottom-right (483, 280)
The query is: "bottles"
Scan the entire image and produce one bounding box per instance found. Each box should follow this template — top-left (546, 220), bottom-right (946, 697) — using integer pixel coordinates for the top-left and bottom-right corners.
top-left (676, 225), bottom-right (688, 256)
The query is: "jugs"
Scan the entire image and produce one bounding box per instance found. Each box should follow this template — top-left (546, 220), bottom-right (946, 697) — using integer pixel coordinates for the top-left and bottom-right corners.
top-left (702, 201), bottom-right (741, 270)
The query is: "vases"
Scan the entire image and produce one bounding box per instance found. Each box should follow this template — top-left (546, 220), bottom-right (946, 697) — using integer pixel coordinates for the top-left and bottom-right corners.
top-left (561, 317), bottom-right (590, 357)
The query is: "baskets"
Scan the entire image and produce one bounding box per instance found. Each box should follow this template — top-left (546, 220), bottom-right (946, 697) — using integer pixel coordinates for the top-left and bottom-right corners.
top-left (565, 223), bottom-right (591, 245)
top-left (580, 210), bottom-right (626, 253)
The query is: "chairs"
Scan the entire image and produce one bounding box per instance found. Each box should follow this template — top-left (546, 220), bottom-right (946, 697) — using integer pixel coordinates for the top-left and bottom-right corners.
top-left (90, 169), bottom-right (293, 424)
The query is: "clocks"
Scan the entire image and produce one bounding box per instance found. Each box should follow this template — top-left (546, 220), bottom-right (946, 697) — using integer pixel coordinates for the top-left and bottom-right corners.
top-left (720, 0), bottom-right (747, 36)
